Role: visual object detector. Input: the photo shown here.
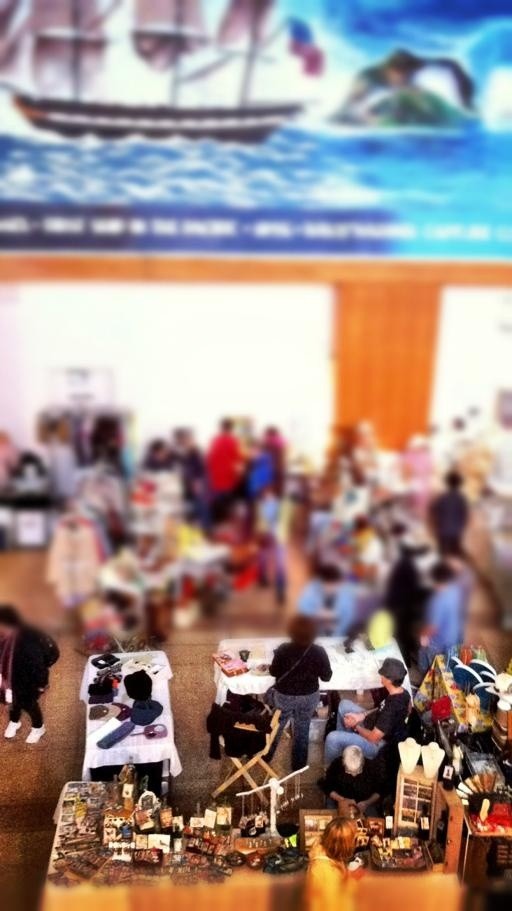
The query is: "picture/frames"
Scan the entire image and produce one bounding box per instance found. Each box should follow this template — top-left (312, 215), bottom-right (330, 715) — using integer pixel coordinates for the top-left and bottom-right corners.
top-left (299, 809), bottom-right (336, 854)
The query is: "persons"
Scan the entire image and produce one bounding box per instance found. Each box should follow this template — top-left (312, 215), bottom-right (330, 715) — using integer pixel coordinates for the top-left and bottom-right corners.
top-left (301, 815), bottom-right (367, 911)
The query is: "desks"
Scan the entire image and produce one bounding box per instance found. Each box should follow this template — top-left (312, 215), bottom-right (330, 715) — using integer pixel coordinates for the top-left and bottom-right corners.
top-left (214, 638), bottom-right (411, 704)
top-left (83, 651), bottom-right (169, 798)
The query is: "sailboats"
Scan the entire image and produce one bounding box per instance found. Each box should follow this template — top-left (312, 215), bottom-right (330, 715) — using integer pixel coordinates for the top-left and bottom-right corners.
top-left (0, 0), bottom-right (307, 144)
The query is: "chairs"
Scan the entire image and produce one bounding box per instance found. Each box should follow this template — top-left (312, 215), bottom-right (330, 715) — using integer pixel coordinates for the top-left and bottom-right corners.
top-left (208, 707), bottom-right (286, 806)
